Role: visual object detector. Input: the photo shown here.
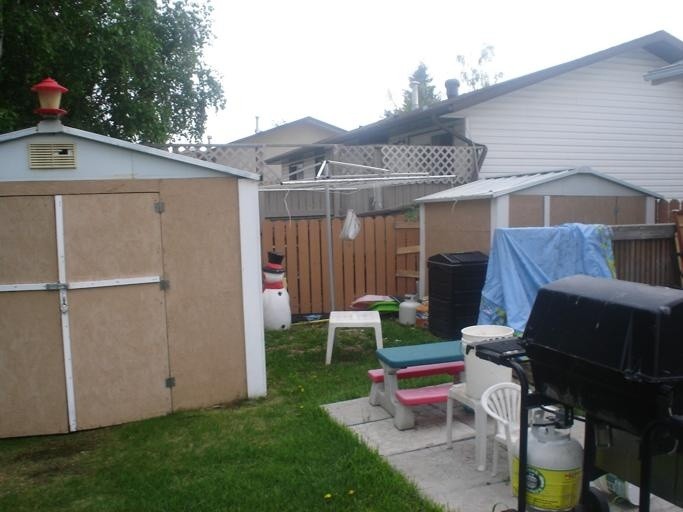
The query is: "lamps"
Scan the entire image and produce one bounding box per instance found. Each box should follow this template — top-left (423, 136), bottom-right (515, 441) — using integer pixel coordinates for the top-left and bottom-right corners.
top-left (32, 75), bottom-right (67, 120)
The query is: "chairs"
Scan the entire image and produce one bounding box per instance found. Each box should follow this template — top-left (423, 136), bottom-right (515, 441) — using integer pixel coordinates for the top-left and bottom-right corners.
top-left (484, 381), bottom-right (534, 482)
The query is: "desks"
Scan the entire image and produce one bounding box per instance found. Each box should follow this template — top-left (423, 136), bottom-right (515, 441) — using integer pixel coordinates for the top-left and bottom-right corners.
top-left (503, 354), bottom-right (682, 511)
top-left (375, 339), bottom-right (466, 431)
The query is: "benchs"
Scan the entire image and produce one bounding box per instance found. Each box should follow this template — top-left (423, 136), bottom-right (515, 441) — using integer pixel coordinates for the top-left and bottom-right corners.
top-left (366, 359), bottom-right (466, 408)
top-left (392, 380), bottom-right (466, 428)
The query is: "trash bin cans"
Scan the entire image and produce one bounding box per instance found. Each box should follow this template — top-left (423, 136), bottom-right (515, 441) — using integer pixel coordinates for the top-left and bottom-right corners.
top-left (427, 251), bottom-right (489, 340)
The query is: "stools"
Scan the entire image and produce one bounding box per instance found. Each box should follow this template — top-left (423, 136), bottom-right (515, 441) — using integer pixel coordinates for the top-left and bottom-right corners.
top-left (325, 310), bottom-right (385, 363)
top-left (446, 382), bottom-right (508, 471)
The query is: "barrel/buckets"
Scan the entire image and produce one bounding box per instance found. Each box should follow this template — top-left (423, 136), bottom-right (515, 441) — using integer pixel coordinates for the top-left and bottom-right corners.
top-left (460, 324), bottom-right (516, 401)
